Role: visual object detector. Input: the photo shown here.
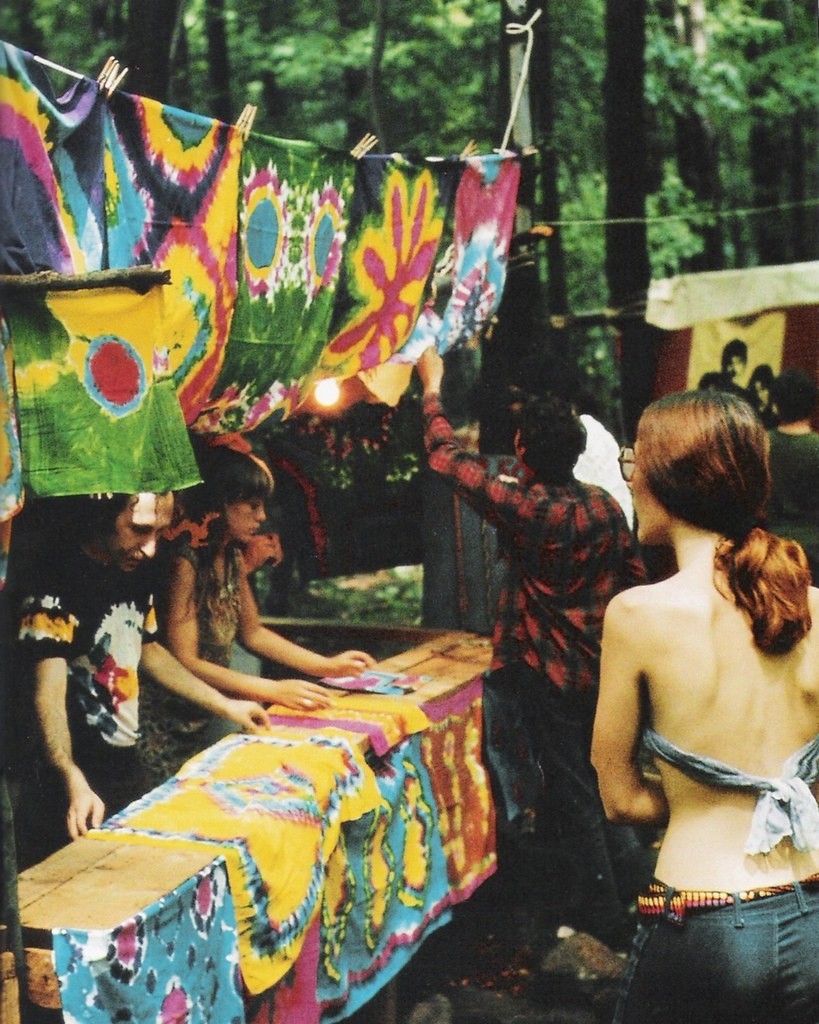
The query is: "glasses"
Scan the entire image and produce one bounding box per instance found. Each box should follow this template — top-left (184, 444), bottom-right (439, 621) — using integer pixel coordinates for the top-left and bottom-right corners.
top-left (618, 445), bottom-right (638, 481)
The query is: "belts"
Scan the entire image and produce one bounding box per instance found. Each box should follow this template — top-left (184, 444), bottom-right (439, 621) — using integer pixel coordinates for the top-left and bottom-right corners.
top-left (638, 876), bottom-right (819, 928)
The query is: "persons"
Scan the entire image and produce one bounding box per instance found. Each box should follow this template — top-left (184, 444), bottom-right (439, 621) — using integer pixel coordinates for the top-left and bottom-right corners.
top-left (0, 446), bottom-right (376, 857)
top-left (419, 347), bottom-right (819, 964)
top-left (589, 391), bottom-right (819, 1024)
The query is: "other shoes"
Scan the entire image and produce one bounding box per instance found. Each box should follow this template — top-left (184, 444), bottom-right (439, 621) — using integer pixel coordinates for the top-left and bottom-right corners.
top-left (596, 924), bottom-right (637, 952)
